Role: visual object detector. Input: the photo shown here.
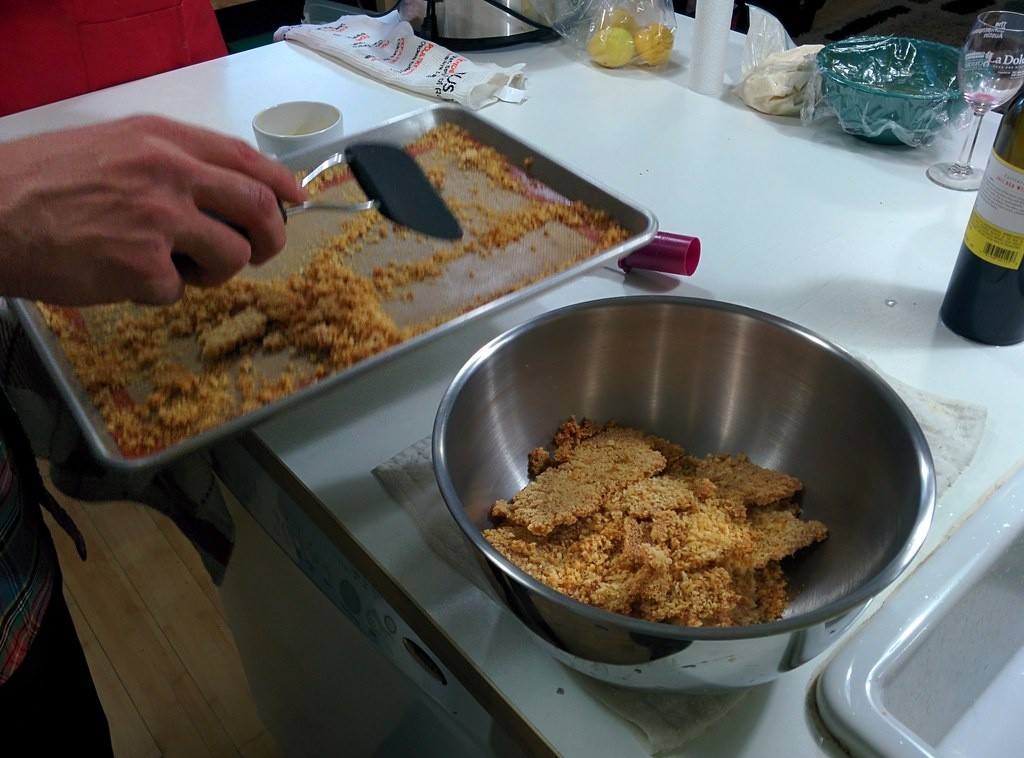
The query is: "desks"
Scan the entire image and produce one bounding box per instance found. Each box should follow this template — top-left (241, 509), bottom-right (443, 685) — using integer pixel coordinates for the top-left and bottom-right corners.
top-left (0, 12), bottom-right (1024, 758)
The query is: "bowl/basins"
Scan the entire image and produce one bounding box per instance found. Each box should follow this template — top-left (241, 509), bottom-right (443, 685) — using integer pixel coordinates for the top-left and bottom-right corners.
top-left (432, 296), bottom-right (936, 690)
top-left (815, 34), bottom-right (969, 146)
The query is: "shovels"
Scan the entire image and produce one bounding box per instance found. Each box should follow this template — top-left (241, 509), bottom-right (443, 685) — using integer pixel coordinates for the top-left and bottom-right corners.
top-left (278, 140), bottom-right (465, 239)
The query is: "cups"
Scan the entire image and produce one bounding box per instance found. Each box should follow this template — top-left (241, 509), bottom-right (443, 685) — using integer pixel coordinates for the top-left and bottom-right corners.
top-left (252, 100), bottom-right (343, 156)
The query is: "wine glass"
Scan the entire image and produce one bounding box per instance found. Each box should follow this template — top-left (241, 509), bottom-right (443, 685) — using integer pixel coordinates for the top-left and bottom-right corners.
top-left (926, 9), bottom-right (1024, 191)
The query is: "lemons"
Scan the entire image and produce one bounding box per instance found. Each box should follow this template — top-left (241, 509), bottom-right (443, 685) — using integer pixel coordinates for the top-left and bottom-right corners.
top-left (588, 8), bottom-right (675, 68)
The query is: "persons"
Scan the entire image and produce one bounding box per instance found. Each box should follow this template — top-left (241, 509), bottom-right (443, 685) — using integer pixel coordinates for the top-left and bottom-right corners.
top-left (0, 112), bottom-right (313, 758)
top-left (0, 0), bottom-right (228, 120)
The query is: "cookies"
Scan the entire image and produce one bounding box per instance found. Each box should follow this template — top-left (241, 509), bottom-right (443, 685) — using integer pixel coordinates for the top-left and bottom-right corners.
top-left (481, 429), bottom-right (826, 616)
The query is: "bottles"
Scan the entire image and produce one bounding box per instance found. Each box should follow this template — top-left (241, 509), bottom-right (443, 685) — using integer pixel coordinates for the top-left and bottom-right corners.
top-left (939, 84), bottom-right (1024, 347)
